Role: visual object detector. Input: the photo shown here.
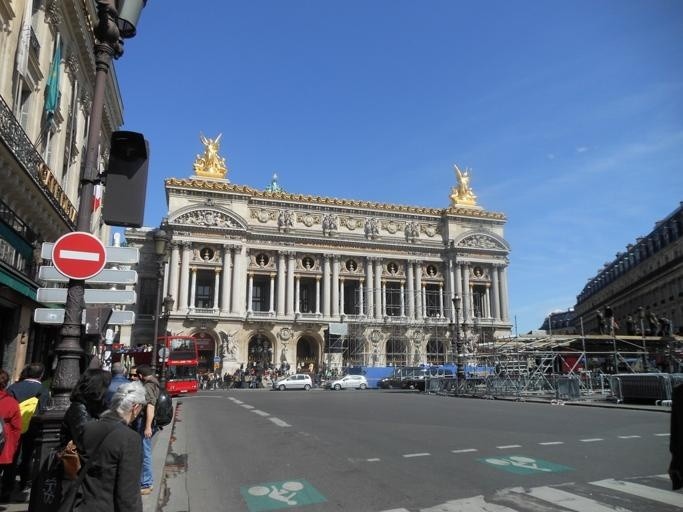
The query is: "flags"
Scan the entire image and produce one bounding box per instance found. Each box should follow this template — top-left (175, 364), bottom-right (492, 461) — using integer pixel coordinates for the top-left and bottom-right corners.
top-left (94, 152), bottom-right (101, 230)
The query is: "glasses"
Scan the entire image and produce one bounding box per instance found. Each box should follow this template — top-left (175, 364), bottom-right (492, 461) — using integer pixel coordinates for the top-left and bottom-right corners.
top-left (129, 373), bottom-right (138, 377)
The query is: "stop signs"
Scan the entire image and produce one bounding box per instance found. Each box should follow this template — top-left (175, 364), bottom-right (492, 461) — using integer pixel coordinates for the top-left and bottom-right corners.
top-left (52, 231), bottom-right (107, 280)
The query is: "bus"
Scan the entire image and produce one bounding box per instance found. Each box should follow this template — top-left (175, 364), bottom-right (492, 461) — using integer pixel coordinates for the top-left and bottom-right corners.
top-left (112, 335), bottom-right (200, 397)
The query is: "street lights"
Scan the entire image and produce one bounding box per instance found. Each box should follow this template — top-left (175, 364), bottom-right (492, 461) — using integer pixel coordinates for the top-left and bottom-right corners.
top-left (149, 227), bottom-right (174, 422)
top-left (29, 0), bottom-right (148, 512)
top-left (451, 293), bottom-right (464, 377)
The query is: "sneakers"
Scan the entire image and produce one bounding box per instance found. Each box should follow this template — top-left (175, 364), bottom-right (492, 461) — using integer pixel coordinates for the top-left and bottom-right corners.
top-left (140, 485), bottom-right (153, 495)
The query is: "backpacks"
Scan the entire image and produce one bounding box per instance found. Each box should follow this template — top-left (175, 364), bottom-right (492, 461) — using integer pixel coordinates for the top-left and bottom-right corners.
top-left (144, 380), bottom-right (173, 426)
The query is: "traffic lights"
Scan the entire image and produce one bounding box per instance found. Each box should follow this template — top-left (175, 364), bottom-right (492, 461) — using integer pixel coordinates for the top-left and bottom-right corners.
top-left (86, 306), bottom-right (113, 342)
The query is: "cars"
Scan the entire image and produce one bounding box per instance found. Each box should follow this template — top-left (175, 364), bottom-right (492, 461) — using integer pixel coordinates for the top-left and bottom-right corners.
top-left (376, 366), bottom-right (429, 392)
top-left (330, 375), bottom-right (368, 391)
top-left (273, 373), bottom-right (312, 391)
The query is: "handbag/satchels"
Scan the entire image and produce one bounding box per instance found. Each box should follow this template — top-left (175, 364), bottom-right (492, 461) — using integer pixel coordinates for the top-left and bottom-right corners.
top-left (19, 396), bottom-right (39, 434)
top-left (31, 471), bottom-right (80, 512)
top-left (0, 417), bottom-right (5, 453)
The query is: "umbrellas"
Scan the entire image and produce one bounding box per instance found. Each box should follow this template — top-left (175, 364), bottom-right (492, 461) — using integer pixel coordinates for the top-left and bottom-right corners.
top-left (43, 31), bottom-right (61, 164)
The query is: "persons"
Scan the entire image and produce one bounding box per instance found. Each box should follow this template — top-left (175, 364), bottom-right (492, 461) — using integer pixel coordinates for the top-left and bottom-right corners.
top-left (0, 362), bottom-right (160, 512)
top-left (197, 362), bottom-right (331, 389)
